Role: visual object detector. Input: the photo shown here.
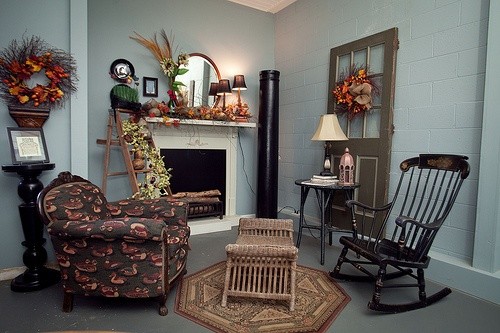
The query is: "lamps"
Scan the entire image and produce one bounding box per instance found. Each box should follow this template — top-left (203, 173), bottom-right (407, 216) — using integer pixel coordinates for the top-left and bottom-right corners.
top-left (232, 74), bottom-right (248, 122)
top-left (209, 82), bottom-right (221, 102)
top-left (309, 114), bottom-right (349, 180)
top-left (217, 79), bottom-right (231, 110)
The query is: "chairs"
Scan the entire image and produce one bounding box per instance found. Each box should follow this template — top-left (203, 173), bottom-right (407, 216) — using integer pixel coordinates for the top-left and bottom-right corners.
top-left (223, 219), bottom-right (298, 312)
top-left (327, 150), bottom-right (474, 314)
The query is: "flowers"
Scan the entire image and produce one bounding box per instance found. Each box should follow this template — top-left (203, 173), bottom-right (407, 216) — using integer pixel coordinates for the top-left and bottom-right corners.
top-left (0, 37), bottom-right (77, 109)
top-left (144, 30), bottom-right (188, 91)
top-left (333, 67), bottom-right (378, 115)
top-left (120, 117), bottom-right (170, 204)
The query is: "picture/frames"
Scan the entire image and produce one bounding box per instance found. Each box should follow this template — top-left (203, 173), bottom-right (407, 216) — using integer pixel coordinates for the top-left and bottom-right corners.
top-left (143, 75), bottom-right (159, 96)
top-left (7, 126), bottom-right (50, 163)
top-left (110, 58), bottom-right (138, 83)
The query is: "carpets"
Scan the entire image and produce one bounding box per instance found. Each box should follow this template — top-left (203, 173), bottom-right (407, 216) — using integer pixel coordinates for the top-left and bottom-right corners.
top-left (174, 259), bottom-right (352, 332)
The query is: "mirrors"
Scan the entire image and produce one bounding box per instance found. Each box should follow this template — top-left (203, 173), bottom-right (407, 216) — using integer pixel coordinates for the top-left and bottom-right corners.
top-left (176, 52), bottom-right (220, 111)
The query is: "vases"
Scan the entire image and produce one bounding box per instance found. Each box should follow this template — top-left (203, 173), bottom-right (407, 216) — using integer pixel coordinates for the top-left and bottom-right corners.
top-left (167, 90), bottom-right (179, 115)
top-left (7, 98), bottom-right (51, 128)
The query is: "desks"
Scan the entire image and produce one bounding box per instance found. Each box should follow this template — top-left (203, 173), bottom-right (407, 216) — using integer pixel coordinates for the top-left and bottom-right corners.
top-left (294, 179), bottom-right (361, 265)
top-left (2, 161), bottom-right (61, 293)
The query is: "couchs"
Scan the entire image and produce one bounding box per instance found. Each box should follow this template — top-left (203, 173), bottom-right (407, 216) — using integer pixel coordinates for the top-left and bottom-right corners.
top-left (38, 173), bottom-right (192, 316)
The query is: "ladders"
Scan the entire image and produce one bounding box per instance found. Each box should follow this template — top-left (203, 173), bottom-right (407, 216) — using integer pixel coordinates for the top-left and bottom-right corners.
top-left (100, 108), bottom-right (173, 200)
